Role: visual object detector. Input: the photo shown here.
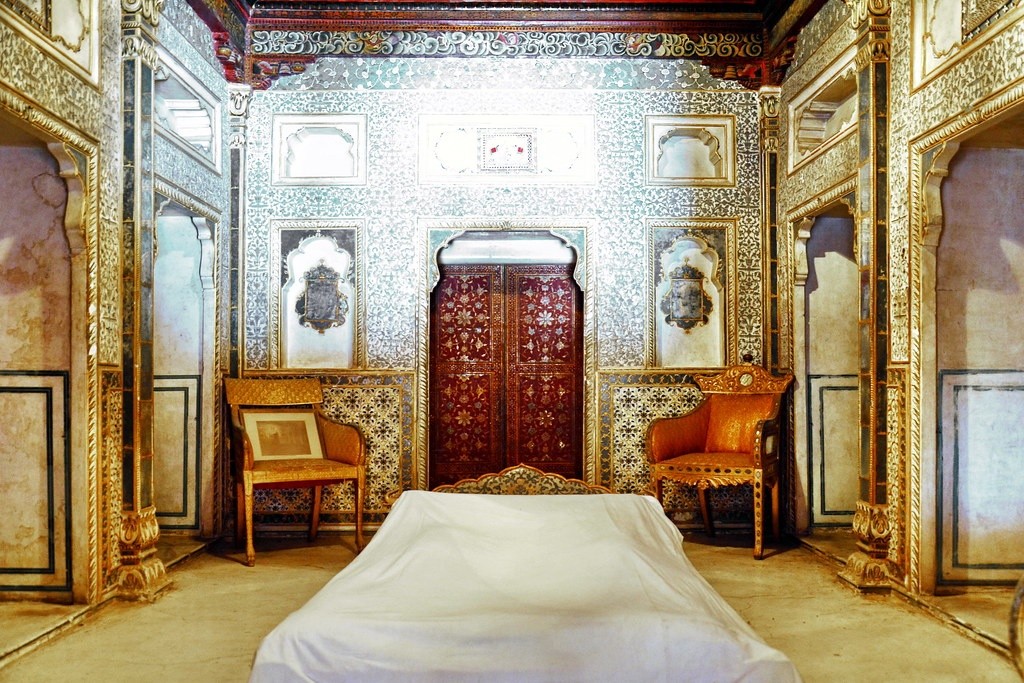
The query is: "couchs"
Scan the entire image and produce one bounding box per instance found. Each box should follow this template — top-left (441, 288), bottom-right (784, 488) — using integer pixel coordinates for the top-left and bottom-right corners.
top-left (238, 462), bottom-right (800, 683)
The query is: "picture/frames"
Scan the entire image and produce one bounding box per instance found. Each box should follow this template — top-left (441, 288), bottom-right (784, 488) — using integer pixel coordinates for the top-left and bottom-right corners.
top-left (235, 406), bottom-right (332, 465)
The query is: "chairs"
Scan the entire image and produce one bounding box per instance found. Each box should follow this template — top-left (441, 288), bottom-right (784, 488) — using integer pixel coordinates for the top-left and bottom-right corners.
top-left (636, 354), bottom-right (798, 562)
top-left (218, 374), bottom-right (369, 571)
